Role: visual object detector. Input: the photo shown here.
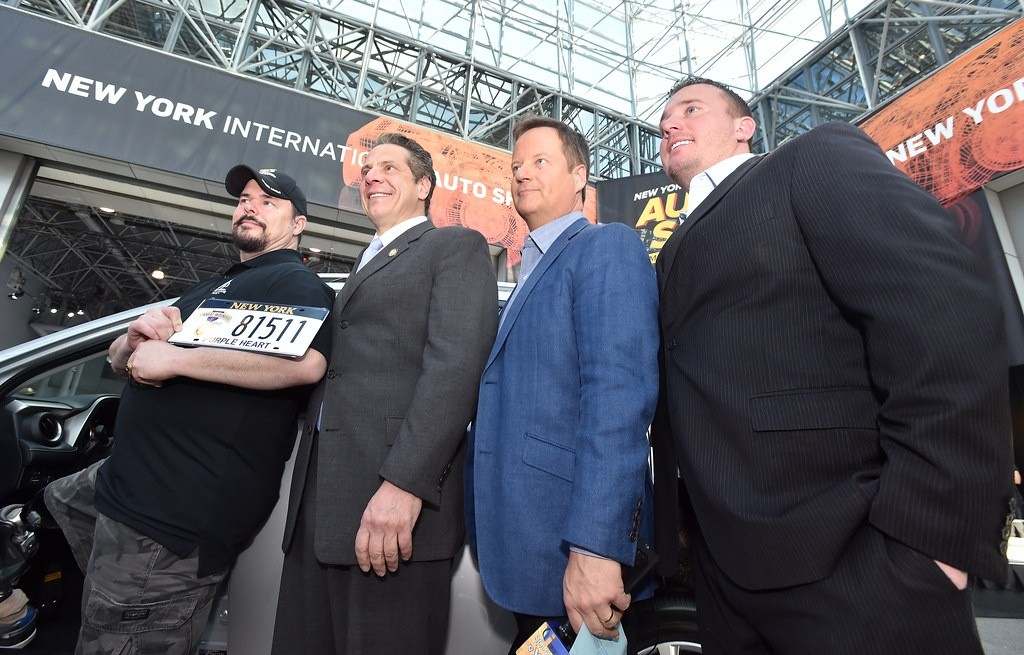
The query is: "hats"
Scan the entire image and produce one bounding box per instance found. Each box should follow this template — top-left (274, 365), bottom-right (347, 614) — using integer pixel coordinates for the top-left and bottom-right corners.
top-left (225, 165), bottom-right (308, 221)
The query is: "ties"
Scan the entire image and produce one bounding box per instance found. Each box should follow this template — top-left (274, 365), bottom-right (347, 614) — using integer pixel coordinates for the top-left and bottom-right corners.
top-left (355, 237), bottom-right (383, 275)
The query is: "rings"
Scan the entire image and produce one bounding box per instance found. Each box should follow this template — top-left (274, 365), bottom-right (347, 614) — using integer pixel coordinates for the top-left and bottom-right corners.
top-left (600, 610), bottom-right (613, 623)
top-left (126, 364), bottom-right (132, 371)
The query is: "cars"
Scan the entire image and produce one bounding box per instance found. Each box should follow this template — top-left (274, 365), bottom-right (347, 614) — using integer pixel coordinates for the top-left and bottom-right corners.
top-left (1, 272), bottom-right (700, 655)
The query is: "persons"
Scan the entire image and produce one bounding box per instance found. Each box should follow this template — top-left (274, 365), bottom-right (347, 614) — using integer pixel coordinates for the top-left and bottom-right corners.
top-left (270, 131), bottom-right (499, 655)
top-left (652, 76), bottom-right (1015, 655)
top-left (45, 164), bottom-right (336, 655)
top-left (465, 113), bottom-right (660, 655)
